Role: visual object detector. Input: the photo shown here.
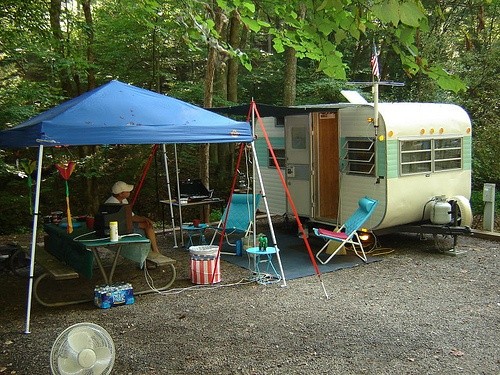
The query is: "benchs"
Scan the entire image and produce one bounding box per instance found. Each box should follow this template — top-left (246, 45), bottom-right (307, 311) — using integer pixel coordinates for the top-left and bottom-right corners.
top-left (146, 251), bottom-right (177, 292)
top-left (12, 244), bottom-right (79, 305)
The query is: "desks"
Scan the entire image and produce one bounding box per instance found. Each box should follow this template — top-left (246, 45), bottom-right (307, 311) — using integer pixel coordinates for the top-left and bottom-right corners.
top-left (246, 247), bottom-right (281, 284)
top-left (44, 223), bottom-right (151, 301)
top-left (160, 199), bottom-right (225, 247)
top-left (182, 224), bottom-right (209, 250)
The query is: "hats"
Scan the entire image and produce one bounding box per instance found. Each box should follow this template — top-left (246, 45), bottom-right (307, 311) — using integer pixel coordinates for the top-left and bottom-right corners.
top-left (112, 181), bottom-right (134, 194)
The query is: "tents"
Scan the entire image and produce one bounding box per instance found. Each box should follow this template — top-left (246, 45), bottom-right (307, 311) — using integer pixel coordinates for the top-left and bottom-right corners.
top-left (0, 79), bottom-right (286, 333)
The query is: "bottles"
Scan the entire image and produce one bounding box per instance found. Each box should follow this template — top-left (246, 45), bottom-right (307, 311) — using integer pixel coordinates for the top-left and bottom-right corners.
top-left (93, 280), bottom-right (135, 308)
top-left (259, 235), bottom-right (268, 251)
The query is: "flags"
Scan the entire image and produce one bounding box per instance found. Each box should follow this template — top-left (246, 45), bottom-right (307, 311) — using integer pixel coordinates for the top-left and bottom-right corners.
top-left (371, 43), bottom-right (379, 78)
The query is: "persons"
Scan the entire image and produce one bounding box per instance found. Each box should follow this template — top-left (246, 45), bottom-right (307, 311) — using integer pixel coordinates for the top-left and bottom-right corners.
top-left (105, 181), bottom-right (159, 253)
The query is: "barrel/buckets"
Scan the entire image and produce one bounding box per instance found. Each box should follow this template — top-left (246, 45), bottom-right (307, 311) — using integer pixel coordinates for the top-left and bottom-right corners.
top-left (51, 211), bottom-right (63, 224)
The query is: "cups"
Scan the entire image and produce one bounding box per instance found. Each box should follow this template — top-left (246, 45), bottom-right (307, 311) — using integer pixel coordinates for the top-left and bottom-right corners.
top-left (87, 216), bottom-right (94, 229)
top-left (193, 219), bottom-right (200, 226)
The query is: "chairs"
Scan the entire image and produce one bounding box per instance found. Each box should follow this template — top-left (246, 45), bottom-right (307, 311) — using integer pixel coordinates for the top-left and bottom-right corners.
top-left (313, 195), bottom-right (378, 264)
top-left (210, 194), bottom-right (262, 255)
